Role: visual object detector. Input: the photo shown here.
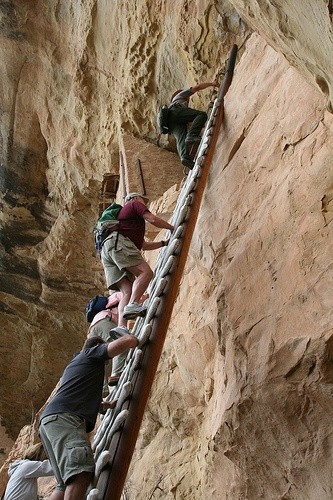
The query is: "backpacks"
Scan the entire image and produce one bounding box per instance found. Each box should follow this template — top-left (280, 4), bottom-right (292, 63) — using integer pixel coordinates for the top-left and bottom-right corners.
top-left (157, 105), bottom-right (172, 134)
top-left (94, 201), bottom-right (125, 251)
top-left (85, 294), bottom-right (118, 323)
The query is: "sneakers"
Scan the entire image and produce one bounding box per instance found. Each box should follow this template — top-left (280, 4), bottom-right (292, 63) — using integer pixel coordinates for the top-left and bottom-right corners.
top-left (109, 325), bottom-right (129, 339)
top-left (123, 301), bottom-right (149, 320)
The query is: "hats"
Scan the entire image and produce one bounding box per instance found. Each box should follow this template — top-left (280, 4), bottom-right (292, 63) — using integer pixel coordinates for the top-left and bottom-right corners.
top-left (170, 90), bottom-right (184, 101)
top-left (124, 192), bottom-right (149, 206)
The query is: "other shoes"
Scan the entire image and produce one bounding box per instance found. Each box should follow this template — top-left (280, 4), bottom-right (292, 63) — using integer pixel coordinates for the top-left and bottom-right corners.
top-left (108, 376), bottom-right (122, 387)
top-left (102, 391), bottom-right (109, 398)
top-left (185, 138), bottom-right (201, 144)
top-left (181, 160), bottom-right (195, 170)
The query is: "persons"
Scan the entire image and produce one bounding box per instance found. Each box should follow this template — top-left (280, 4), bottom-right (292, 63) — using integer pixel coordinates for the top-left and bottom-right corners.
top-left (93, 192), bottom-right (174, 341)
top-left (38, 333), bottom-right (139, 500)
top-left (86, 291), bottom-right (150, 398)
top-left (4, 444), bottom-right (55, 500)
top-left (157, 79), bottom-right (220, 170)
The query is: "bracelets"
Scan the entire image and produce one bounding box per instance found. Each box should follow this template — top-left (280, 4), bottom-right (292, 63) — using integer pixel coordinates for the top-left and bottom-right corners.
top-left (161, 241), bottom-right (164, 247)
